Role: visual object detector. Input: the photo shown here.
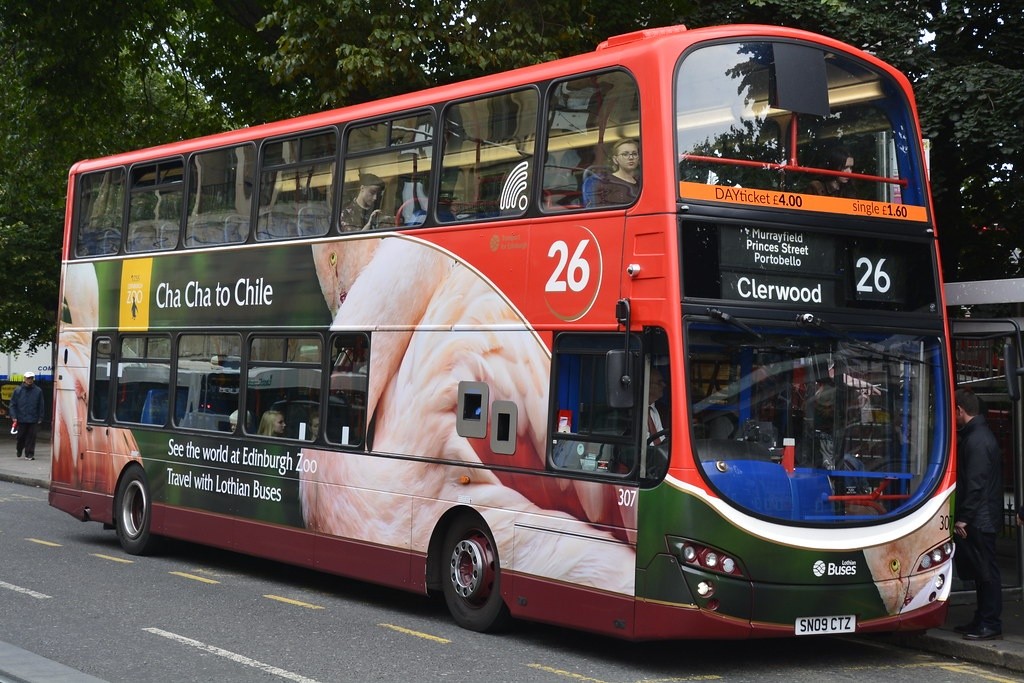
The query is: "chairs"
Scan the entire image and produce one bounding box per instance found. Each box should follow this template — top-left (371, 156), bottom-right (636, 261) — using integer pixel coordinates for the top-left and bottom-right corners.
top-left (71, 163), bottom-right (615, 261)
top-left (81, 359), bottom-right (372, 446)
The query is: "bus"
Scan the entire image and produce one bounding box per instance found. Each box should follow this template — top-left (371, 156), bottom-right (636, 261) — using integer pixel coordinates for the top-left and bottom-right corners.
top-left (48, 23), bottom-right (1023, 642)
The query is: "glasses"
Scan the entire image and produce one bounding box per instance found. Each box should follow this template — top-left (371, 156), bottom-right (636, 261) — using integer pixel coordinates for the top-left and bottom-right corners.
top-left (615, 152), bottom-right (639, 160)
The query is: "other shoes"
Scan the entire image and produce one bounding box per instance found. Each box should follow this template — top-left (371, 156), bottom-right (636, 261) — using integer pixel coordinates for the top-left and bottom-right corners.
top-left (17, 450), bottom-right (22, 457)
top-left (25, 456), bottom-right (34, 460)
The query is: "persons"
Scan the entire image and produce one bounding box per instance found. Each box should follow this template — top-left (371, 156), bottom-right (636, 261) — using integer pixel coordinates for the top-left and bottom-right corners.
top-left (801, 145), bottom-right (855, 197)
top-left (612, 366), bottom-right (671, 471)
top-left (229, 408), bottom-right (253, 433)
top-left (257, 410), bottom-right (286, 437)
top-left (593, 138), bottom-right (643, 207)
top-left (339, 174), bottom-right (386, 232)
top-left (8, 372), bottom-right (44, 461)
top-left (308, 411), bottom-right (320, 441)
top-left (949, 387), bottom-right (1004, 640)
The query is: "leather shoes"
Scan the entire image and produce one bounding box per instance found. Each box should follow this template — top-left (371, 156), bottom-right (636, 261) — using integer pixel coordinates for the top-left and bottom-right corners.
top-left (954, 622), bottom-right (1004, 641)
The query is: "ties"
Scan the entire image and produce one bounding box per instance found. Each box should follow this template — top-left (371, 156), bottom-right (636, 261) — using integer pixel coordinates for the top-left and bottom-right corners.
top-left (648, 406), bottom-right (661, 446)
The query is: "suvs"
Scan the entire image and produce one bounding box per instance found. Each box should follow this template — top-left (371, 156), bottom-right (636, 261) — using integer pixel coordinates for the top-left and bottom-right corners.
top-left (115, 354), bottom-right (368, 446)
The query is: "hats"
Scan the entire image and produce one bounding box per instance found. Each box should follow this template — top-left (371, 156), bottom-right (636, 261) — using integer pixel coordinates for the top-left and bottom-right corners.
top-left (24, 372), bottom-right (35, 378)
